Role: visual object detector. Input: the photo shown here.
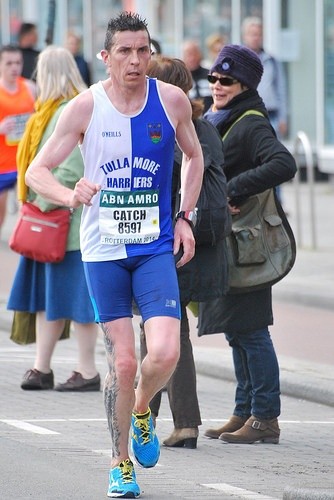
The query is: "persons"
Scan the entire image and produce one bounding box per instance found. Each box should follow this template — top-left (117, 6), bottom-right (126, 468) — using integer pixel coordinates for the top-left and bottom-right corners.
top-left (6, 45), bottom-right (101, 392)
top-left (201, 42), bottom-right (298, 446)
top-left (139, 57), bottom-right (231, 449)
top-left (0, 8), bottom-right (289, 248)
top-left (24, 11), bottom-right (204, 499)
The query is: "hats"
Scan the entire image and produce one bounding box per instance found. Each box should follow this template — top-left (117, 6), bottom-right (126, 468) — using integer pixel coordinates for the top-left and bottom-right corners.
top-left (209, 44), bottom-right (264, 90)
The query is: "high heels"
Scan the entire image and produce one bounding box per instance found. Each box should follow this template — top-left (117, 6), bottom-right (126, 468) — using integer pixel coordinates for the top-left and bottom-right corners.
top-left (163, 429), bottom-right (199, 448)
top-left (151, 413), bottom-right (157, 428)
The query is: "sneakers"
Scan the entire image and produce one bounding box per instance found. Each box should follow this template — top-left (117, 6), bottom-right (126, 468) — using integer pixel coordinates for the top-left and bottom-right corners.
top-left (132, 407), bottom-right (160, 468)
top-left (108, 459), bottom-right (141, 498)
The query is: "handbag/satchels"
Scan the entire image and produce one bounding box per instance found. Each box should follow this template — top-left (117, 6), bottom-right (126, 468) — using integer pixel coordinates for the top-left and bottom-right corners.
top-left (10, 203), bottom-right (70, 263)
top-left (228, 188), bottom-right (297, 296)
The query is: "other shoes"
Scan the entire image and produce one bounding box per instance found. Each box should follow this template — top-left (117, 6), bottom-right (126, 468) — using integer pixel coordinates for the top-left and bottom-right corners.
top-left (220, 416), bottom-right (280, 443)
top-left (204, 415), bottom-right (247, 438)
top-left (21, 369), bottom-right (53, 390)
top-left (54, 371), bottom-right (100, 392)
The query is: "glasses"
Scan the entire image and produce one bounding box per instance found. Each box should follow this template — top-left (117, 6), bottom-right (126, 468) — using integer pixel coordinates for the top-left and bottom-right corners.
top-left (208, 74), bottom-right (240, 86)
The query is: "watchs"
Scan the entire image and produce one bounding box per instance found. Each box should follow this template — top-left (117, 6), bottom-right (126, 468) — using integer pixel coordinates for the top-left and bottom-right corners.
top-left (176, 209), bottom-right (198, 229)
